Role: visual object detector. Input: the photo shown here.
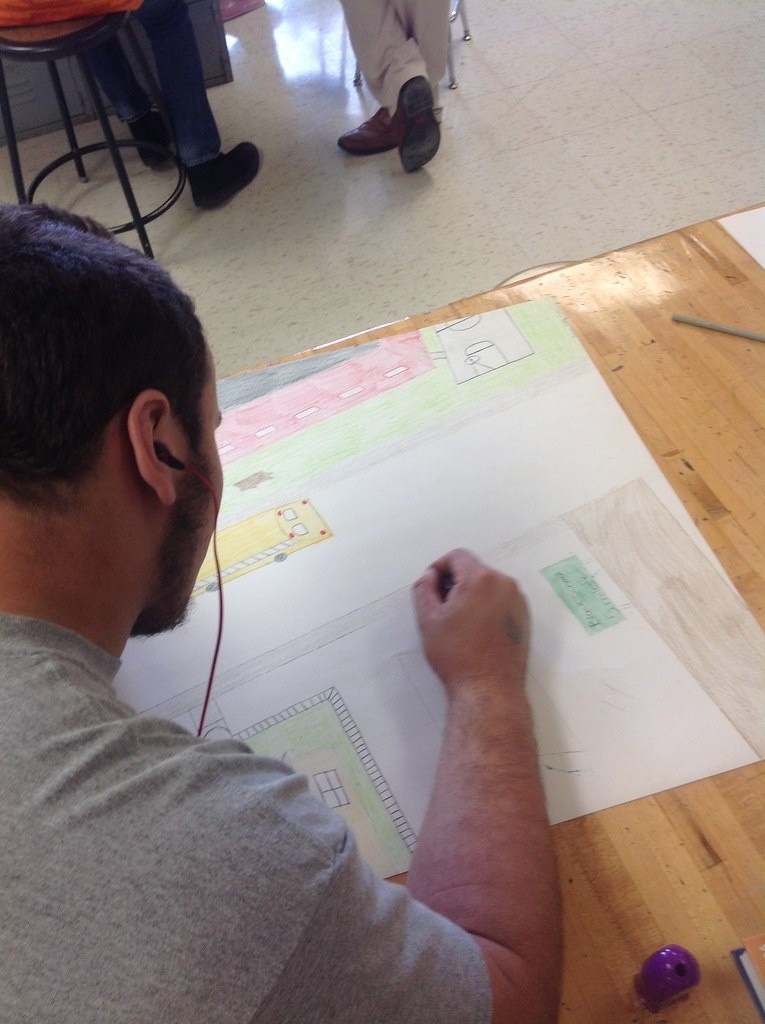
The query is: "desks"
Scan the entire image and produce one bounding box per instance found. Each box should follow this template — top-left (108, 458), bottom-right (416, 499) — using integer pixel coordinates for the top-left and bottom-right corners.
top-left (114, 202), bottom-right (765, 1024)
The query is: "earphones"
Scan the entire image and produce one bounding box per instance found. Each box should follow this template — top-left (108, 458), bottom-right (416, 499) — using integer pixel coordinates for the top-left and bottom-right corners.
top-left (151, 441), bottom-right (185, 471)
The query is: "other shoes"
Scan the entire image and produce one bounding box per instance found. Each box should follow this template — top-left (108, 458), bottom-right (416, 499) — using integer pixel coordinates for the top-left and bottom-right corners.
top-left (189, 142), bottom-right (259, 209)
top-left (130, 113), bottom-right (170, 167)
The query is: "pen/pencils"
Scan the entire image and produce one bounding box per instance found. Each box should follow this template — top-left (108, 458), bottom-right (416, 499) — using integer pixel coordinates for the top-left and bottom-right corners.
top-left (439, 581), bottom-right (453, 589)
top-left (732, 947), bottom-right (765, 1022)
top-left (671, 312), bottom-right (765, 346)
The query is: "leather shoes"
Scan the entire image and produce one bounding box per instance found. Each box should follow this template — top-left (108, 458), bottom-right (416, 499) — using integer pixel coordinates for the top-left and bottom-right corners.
top-left (397, 74), bottom-right (440, 172)
top-left (338, 106), bottom-right (399, 155)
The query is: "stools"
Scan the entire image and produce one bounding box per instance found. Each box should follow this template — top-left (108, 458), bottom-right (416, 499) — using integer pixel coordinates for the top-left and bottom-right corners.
top-left (0, 9), bottom-right (187, 260)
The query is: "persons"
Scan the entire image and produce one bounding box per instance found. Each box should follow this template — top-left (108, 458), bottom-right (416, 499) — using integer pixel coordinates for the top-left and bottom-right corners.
top-left (0, 200), bottom-right (568, 1024)
top-left (330, 0), bottom-right (452, 173)
top-left (0, 0), bottom-right (259, 209)
